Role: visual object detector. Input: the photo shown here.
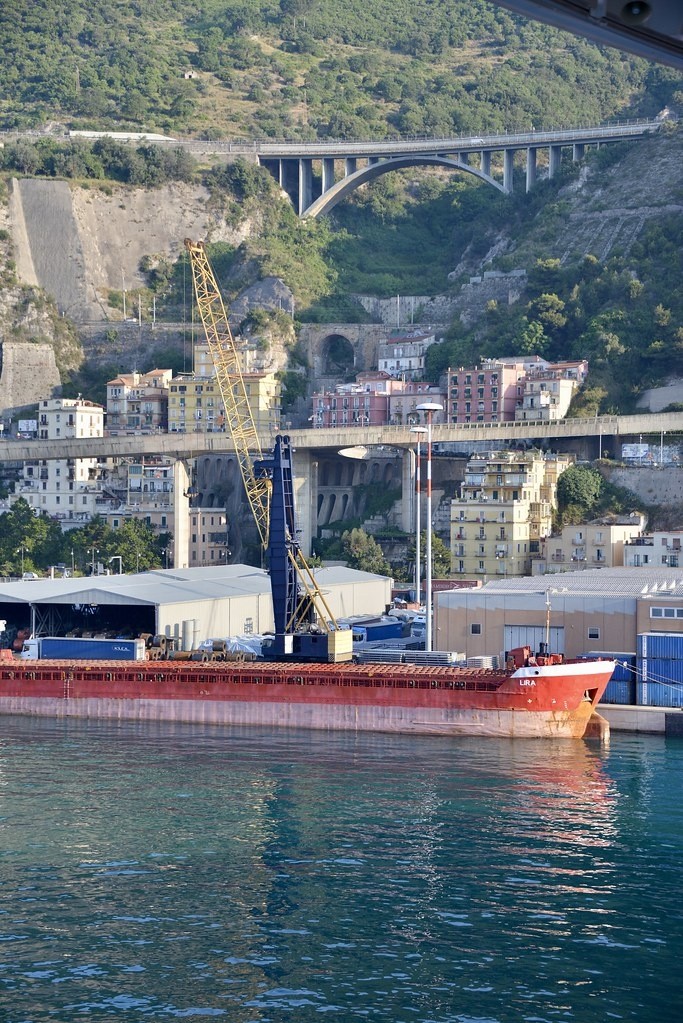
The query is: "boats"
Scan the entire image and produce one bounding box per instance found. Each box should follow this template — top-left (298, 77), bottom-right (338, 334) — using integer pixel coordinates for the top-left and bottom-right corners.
top-left (0, 238), bottom-right (621, 739)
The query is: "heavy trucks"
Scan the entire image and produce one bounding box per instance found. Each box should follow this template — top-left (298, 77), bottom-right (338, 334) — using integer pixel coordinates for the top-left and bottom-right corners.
top-left (409, 616), bottom-right (433, 640)
top-left (20, 637), bottom-right (146, 662)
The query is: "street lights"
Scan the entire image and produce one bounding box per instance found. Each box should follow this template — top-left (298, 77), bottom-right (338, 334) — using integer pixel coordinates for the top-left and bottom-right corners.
top-left (409, 426), bottom-right (429, 608)
top-left (86, 545), bottom-right (99, 576)
top-left (415, 402), bottom-right (443, 650)
top-left (221, 548), bottom-right (232, 565)
top-left (16, 544), bottom-right (29, 577)
top-left (161, 547), bottom-right (173, 568)
